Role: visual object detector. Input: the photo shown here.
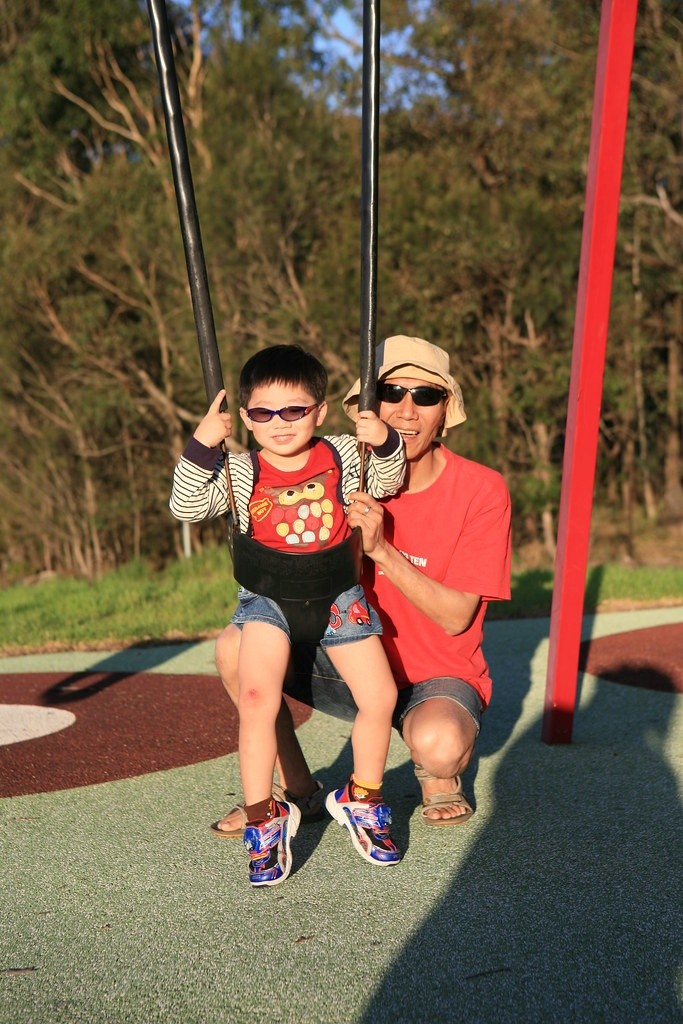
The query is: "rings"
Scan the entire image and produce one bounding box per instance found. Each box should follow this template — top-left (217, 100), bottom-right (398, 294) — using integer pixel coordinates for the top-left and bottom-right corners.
top-left (363, 506), bottom-right (370, 516)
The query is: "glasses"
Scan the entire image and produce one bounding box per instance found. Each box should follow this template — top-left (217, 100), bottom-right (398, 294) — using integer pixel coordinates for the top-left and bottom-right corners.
top-left (376, 383), bottom-right (447, 406)
top-left (246, 403), bottom-right (319, 423)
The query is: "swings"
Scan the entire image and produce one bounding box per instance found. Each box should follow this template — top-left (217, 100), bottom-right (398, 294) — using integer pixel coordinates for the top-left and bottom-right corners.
top-left (146, 0), bottom-right (381, 648)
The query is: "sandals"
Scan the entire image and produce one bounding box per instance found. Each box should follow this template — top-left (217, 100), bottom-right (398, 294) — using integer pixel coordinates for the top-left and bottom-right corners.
top-left (209, 779), bottom-right (325, 837)
top-left (414, 763), bottom-right (474, 827)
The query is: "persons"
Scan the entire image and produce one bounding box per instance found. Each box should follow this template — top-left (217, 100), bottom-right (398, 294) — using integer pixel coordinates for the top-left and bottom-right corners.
top-left (209, 336), bottom-right (512, 836)
top-left (168, 345), bottom-right (402, 887)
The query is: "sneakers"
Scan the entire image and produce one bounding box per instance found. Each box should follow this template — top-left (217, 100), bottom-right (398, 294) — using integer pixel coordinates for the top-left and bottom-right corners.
top-left (324, 774), bottom-right (401, 867)
top-left (242, 797), bottom-right (302, 888)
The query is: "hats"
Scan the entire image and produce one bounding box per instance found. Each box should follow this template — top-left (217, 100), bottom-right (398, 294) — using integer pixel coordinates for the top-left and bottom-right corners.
top-left (341, 335), bottom-right (467, 439)
